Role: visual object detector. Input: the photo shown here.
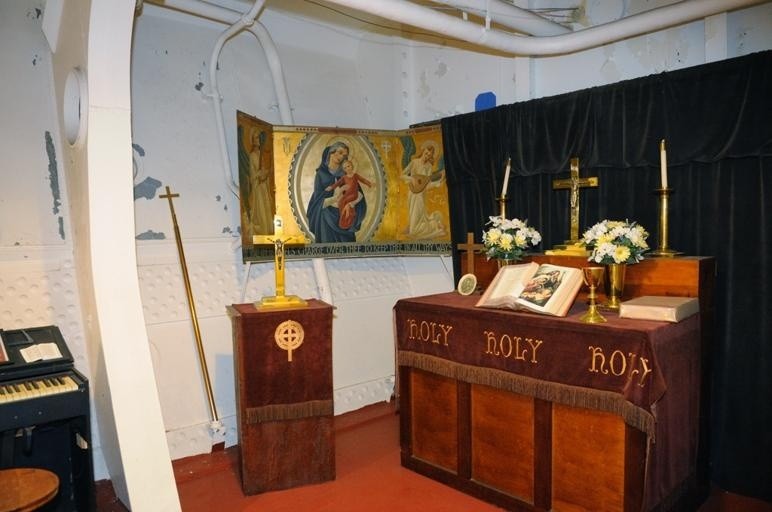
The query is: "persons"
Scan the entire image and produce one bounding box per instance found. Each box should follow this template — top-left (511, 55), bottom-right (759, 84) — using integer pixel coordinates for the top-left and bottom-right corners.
top-left (557, 177), bottom-right (590, 208)
top-left (325, 160), bottom-right (374, 229)
top-left (307, 142), bottom-right (366, 243)
top-left (268, 238), bottom-right (292, 270)
top-left (248, 126), bottom-right (274, 235)
top-left (401, 145), bottom-right (449, 240)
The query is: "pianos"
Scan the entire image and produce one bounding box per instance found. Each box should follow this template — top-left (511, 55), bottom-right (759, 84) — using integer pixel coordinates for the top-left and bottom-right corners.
top-left (0, 326), bottom-right (97, 512)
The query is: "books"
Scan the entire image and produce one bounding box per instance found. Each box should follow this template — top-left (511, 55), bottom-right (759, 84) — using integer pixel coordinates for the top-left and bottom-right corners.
top-left (19, 342), bottom-right (63, 363)
top-left (475, 261), bottom-right (584, 317)
top-left (619, 296), bottom-right (700, 322)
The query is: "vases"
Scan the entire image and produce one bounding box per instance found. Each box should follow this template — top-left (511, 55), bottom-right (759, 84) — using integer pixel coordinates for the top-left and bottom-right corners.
top-left (496, 258), bottom-right (626, 311)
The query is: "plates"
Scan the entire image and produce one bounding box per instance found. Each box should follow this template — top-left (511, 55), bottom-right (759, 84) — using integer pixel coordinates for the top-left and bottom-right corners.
top-left (458, 274), bottom-right (477, 295)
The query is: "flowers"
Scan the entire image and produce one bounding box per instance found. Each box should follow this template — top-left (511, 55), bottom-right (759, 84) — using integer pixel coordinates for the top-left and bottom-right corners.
top-left (481, 215), bottom-right (651, 267)
